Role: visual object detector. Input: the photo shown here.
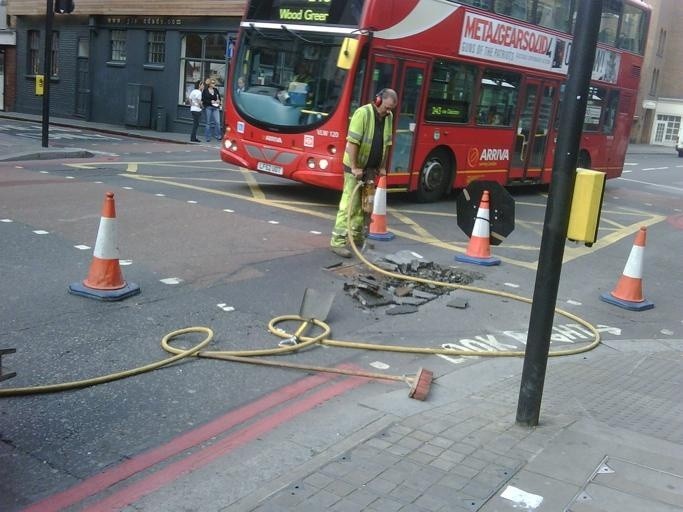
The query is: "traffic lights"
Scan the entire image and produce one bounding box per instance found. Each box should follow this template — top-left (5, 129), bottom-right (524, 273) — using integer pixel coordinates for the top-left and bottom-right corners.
top-left (53, 0), bottom-right (75, 13)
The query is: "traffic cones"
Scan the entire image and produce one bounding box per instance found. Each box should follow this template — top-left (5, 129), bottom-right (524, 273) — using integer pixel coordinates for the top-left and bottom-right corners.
top-left (599, 227), bottom-right (656, 311)
top-left (453, 190), bottom-right (500, 265)
top-left (367, 176), bottom-right (396, 241)
top-left (67, 190), bottom-right (140, 302)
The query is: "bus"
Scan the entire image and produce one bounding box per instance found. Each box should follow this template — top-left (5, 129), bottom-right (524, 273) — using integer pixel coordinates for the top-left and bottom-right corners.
top-left (218, 0), bottom-right (654, 203)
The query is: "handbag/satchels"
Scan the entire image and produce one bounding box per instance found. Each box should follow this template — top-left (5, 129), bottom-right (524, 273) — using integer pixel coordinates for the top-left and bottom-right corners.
top-left (212, 100), bottom-right (219, 107)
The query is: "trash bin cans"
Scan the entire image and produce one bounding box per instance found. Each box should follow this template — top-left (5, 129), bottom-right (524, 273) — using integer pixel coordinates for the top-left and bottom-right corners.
top-left (156, 106), bottom-right (166, 132)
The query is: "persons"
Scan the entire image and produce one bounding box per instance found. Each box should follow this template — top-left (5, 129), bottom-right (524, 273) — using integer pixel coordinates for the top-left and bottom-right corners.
top-left (237, 76), bottom-right (249, 93)
top-left (330, 85), bottom-right (399, 258)
top-left (188, 80), bottom-right (204, 142)
top-left (202, 77), bottom-right (222, 142)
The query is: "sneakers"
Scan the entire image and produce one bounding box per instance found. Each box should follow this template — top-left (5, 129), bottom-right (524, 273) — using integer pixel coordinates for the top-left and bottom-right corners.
top-left (328, 245), bottom-right (352, 258)
top-left (354, 240), bottom-right (375, 249)
